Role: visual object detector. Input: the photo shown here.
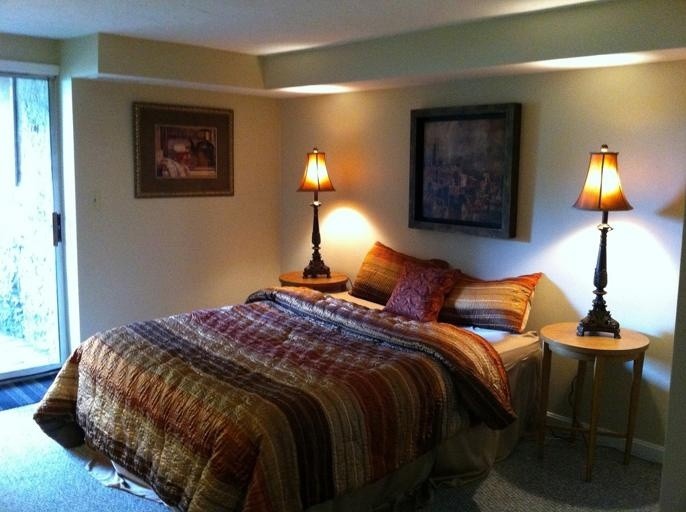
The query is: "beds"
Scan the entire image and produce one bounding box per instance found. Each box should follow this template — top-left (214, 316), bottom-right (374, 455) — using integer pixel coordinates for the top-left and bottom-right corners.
top-left (32, 291), bottom-right (544, 512)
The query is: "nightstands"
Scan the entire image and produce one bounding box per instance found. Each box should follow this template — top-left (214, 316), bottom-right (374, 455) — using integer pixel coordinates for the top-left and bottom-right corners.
top-left (535, 322), bottom-right (650, 483)
top-left (278, 271), bottom-right (349, 293)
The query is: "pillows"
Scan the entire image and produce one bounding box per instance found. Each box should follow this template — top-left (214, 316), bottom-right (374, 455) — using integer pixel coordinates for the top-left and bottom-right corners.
top-left (382, 258), bottom-right (463, 323)
top-left (348, 241), bottom-right (422, 306)
top-left (439, 272), bottom-right (543, 334)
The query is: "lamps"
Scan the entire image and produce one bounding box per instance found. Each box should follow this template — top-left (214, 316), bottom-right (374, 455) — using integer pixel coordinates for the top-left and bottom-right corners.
top-left (572, 144), bottom-right (634, 339)
top-left (296, 147), bottom-right (337, 279)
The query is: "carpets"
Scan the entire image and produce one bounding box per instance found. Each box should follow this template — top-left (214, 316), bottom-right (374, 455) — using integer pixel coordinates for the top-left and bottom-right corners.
top-left (0, 373), bottom-right (59, 411)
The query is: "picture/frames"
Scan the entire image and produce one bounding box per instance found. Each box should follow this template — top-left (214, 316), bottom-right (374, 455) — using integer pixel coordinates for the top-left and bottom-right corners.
top-left (131, 100), bottom-right (235, 200)
top-left (408, 101), bottom-right (521, 240)
